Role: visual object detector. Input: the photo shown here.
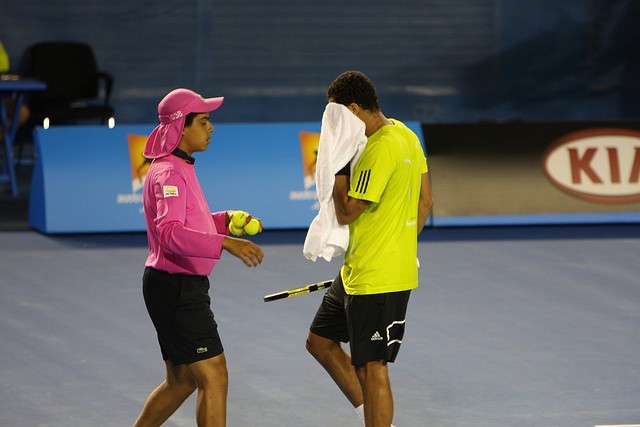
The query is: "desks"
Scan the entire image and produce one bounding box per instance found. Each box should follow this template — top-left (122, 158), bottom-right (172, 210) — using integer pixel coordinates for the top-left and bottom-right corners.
top-left (0, 79), bottom-right (51, 197)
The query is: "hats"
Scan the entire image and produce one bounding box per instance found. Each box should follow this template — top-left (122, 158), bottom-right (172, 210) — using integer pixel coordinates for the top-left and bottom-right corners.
top-left (143, 88), bottom-right (224, 159)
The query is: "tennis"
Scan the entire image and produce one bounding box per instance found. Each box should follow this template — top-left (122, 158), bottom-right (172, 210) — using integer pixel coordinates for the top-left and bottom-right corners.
top-left (244, 218), bottom-right (259, 235)
top-left (231, 211), bottom-right (246, 228)
top-left (229, 222), bottom-right (244, 236)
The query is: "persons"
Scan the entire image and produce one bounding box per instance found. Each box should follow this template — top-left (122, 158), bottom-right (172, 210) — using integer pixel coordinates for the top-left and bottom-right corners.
top-left (304, 71), bottom-right (434, 427)
top-left (0, 41), bottom-right (31, 137)
top-left (131, 89), bottom-right (264, 426)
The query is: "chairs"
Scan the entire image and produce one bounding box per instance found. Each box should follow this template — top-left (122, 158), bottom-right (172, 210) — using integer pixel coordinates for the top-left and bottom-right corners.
top-left (16, 40), bottom-right (115, 166)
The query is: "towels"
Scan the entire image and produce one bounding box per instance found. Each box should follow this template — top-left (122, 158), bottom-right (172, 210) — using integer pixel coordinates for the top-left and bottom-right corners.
top-left (303, 102), bottom-right (368, 262)
